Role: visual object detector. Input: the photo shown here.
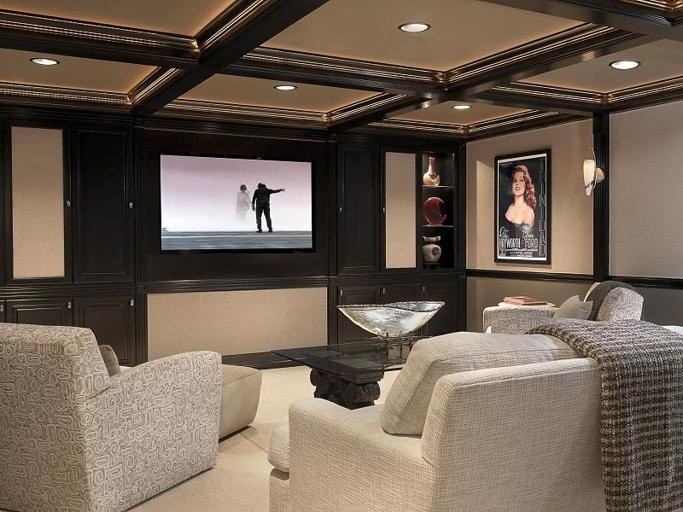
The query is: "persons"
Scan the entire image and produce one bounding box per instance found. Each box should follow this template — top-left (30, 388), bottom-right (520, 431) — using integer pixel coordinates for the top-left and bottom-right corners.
top-left (236, 185), bottom-right (251, 219)
top-left (503, 165), bottom-right (537, 256)
top-left (252, 183), bottom-right (284, 232)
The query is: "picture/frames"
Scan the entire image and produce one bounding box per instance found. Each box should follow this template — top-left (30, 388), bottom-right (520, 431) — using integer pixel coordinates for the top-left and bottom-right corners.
top-left (490, 148), bottom-right (552, 264)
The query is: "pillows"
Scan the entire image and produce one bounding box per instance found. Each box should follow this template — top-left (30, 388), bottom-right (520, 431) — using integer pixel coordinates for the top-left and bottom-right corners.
top-left (552, 295), bottom-right (594, 324)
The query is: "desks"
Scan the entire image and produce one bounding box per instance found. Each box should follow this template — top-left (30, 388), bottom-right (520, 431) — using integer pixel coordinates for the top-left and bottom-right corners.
top-left (483, 303), bottom-right (556, 334)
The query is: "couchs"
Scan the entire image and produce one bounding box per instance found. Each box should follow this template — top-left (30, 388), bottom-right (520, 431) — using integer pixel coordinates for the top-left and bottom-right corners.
top-left (265, 326), bottom-right (683, 511)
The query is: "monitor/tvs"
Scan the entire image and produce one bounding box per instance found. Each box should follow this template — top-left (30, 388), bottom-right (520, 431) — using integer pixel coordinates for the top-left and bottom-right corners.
top-left (156, 151), bottom-right (317, 256)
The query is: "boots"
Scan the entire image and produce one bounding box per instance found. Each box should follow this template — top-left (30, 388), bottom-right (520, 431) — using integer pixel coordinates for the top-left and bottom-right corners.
top-left (268, 228), bottom-right (272, 232)
top-left (256, 229), bottom-right (262, 232)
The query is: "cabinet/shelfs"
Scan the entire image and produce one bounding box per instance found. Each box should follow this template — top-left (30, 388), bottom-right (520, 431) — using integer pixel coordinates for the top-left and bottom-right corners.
top-left (0, 113), bottom-right (138, 368)
top-left (338, 146), bottom-right (464, 343)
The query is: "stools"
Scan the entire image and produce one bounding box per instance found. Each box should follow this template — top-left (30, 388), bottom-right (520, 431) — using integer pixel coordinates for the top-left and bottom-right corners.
top-left (220, 363), bottom-right (259, 438)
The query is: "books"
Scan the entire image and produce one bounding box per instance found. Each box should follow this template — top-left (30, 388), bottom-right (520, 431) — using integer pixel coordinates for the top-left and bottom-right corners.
top-left (504, 295), bottom-right (547, 305)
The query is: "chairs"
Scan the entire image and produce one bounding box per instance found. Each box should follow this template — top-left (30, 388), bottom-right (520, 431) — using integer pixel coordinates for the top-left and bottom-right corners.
top-left (582, 282), bottom-right (643, 326)
top-left (0, 316), bottom-right (221, 511)
